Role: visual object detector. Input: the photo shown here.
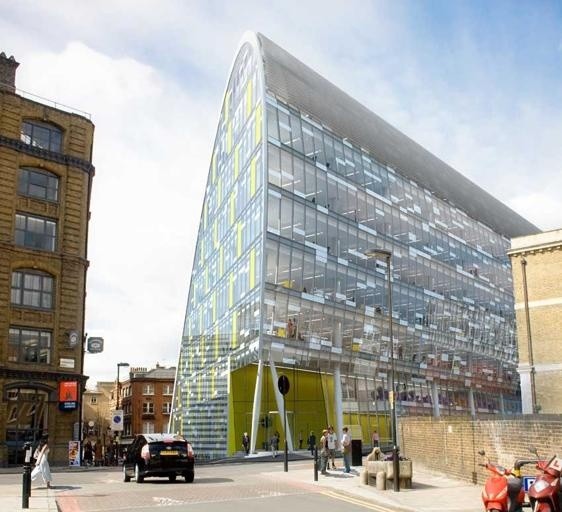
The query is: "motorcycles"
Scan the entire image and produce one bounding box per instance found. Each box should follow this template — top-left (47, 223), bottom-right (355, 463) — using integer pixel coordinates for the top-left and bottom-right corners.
top-left (527, 447), bottom-right (562, 512)
top-left (478, 450), bottom-right (525, 512)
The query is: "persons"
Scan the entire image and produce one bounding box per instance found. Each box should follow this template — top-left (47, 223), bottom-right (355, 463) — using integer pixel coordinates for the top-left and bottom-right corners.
top-left (239, 431), bottom-right (250, 455)
top-left (81, 438), bottom-right (117, 467)
top-left (269, 432), bottom-right (280, 460)
top-left (307, 430), bottom-right (317, 456)
top-left (319, 429), bottom-right (331, 475)
top-left (373, 431), bottom-right (378, 448)
top-left (30, 439), bottom-right (54, 489)
top-left (326, 426), bottom-right (339, 469)
top-left (340, 427), bottom-right (352, 473)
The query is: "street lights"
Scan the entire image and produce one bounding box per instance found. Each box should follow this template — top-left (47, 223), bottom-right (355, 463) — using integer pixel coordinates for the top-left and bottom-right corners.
top-left (116, 363), bottom-right (129, 466)
top-left (364, 249), bottom-right (400, 493)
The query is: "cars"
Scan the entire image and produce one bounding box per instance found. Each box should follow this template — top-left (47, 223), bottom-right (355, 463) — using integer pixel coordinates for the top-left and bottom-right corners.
top-left (122, 434), bottom-right (194, 483)
